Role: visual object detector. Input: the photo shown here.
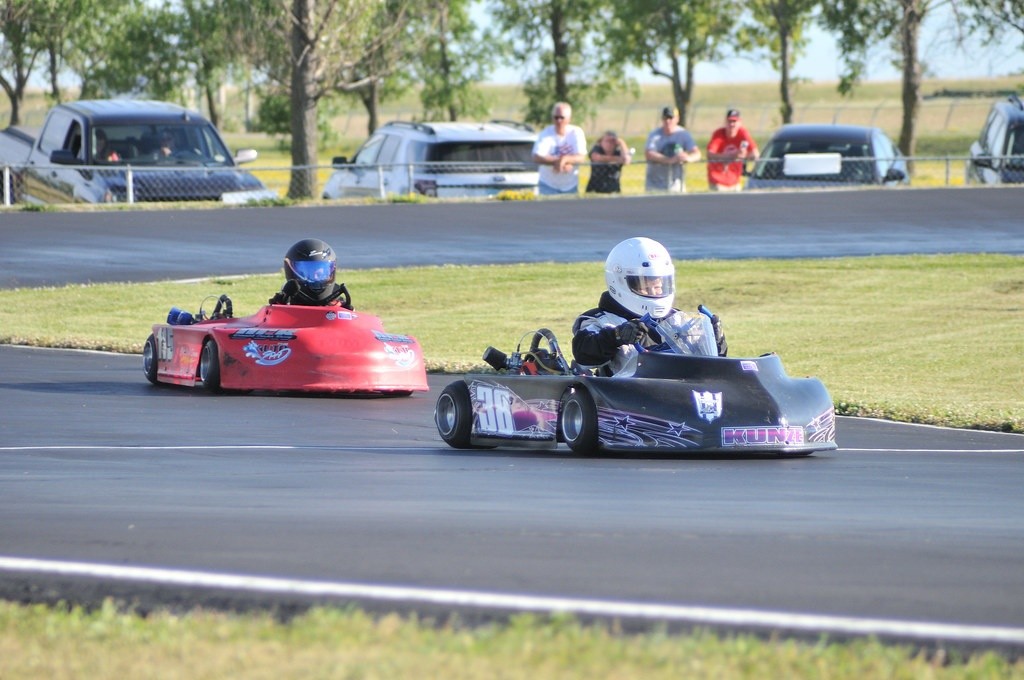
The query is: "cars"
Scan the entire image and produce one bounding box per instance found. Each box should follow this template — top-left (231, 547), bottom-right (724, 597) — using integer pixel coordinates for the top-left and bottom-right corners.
top-left (740, 124), bottom-right (913, 188)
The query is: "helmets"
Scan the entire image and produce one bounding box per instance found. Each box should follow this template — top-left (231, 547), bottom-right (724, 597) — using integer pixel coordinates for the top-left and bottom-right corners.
top-left (284, 239), bottom-right (337, 303)
top-left (605, 236), bottom-right (675, 319)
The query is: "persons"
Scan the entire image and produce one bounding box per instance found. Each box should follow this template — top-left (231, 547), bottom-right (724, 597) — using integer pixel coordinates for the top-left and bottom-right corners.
top-left (269, 238), bottom-right (354, 311)
top-left (532, 102), bottom-right (586, 195)
top-left (706, 110), bottom-right (759, 191)
top-left (586, 131), bottom-right (630, 194)
top-left (152, 127), bottom-right (179, 165)
top-left (77, 129), bottom-right (113, 159)
top-left (645, 106), bottom-right (700, 191)
top-left (573, 237), bottom-right (729, 376)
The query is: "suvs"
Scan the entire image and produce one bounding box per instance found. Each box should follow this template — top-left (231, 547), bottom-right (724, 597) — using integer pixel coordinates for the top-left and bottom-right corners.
top-left (323, 120), bottom-right (541, 201)
top-left (962, 93), bottom-right (1024, 184)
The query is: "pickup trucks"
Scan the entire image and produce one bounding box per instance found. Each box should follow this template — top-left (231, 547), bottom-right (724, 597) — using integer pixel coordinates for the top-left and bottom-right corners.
top-left (0, 100), bottom-right (270, 207)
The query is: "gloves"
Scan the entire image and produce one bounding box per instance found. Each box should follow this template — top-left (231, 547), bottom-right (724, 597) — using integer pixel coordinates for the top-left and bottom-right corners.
top-left (268, 291), bottom-right (288, 304)
top-left (340, 301), bottom-right (354, 311)
top-left (711, 314), bottom-right (725, 345)
top-left (615, 318), bottom-right (649, 347)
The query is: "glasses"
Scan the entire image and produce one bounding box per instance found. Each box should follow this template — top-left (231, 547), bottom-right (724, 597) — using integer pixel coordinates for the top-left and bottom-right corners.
top-left (554, 115), bottom-right (565, 120)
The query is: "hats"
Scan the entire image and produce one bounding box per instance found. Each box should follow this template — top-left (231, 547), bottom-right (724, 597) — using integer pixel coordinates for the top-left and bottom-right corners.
top-left (663, 105), bottom-right (679, 118)
top-left (726, 109), bottom-right (742, 121)
